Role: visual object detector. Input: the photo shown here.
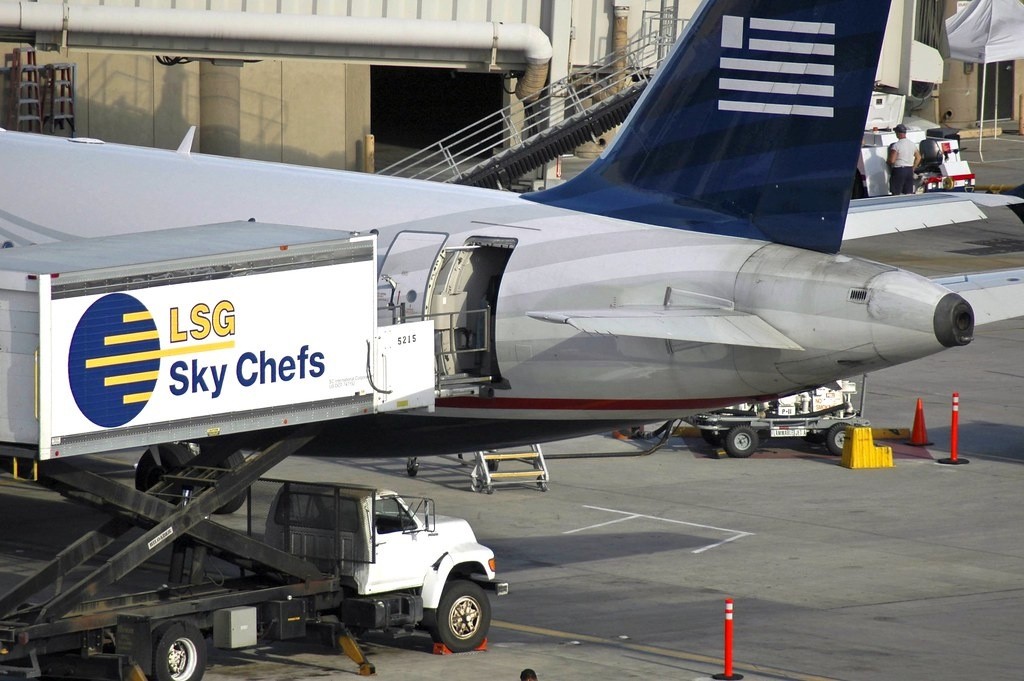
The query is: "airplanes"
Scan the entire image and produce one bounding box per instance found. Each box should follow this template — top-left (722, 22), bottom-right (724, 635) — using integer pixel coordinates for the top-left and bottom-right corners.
top-left (1, 0), bottom-right (977, 516)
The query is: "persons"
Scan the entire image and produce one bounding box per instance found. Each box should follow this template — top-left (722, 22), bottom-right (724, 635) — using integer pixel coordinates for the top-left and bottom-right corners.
top-left (887, 124), bottom-right (921, 195)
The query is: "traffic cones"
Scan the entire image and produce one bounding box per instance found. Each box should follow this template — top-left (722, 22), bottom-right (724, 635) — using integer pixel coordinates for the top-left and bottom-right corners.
top-left (903, 398), bottom-right (936, 446)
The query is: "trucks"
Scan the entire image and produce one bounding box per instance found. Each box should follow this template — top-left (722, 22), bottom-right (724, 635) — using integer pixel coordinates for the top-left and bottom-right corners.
top-left (0, 481), bottom-right (509, 681)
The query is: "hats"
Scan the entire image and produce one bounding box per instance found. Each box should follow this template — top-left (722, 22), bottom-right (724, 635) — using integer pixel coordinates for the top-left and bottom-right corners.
top-left (893, 123), bottom-right (907, 133)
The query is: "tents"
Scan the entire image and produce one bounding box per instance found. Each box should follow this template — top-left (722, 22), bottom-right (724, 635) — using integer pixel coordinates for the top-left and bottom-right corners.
top-left (946, 0), bottom-right (1024, 161)
